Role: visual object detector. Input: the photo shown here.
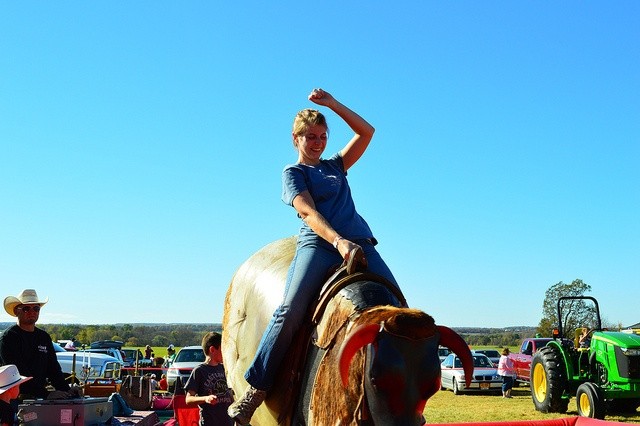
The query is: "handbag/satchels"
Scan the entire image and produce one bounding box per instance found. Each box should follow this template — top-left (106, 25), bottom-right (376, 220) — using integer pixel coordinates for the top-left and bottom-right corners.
top-left (108, 392), bottom-right (133, 416)
top-left (119, 374), bottom-right (152, 410)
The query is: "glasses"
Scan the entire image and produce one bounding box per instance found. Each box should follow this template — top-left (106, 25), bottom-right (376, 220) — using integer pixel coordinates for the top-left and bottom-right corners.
top-left (15, 305), bottom-right (40, 312)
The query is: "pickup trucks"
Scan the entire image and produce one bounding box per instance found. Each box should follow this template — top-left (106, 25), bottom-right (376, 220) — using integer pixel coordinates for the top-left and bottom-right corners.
top-left (50, 341), bottom-right (120, 386)
top-left (507, 338), bottom-right (552, 387)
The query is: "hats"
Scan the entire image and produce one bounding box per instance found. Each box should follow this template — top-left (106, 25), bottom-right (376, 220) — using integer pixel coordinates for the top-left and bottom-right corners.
top-left (0, 364), bottom-right (33, 395)
top-left (3, 289), bottom-right (49, 317)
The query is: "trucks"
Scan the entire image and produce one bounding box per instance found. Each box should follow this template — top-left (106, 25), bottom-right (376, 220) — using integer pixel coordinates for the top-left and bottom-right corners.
top-left (439, 346), bottom-right (450, 362)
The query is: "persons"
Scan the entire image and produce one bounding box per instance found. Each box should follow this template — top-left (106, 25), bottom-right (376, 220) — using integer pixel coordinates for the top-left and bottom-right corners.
top-left (148, 374), bottom-right (158, 391)
top-left (0, 289), bottom-right (79, 400)
top-left (159, 374), bottom-right (168, 390)
top-left (497, 347), bottom-right (517, 399)
top-left (0, 363), bottom-right (34, 426)
top-left (117, 346), bottom-right (126, 362)
top-left (144, 344), bottom-right (152, 359)
top-left (226, 86), bottom-right (410, 426)
top-left (184, 332), bottom-right (232, 426)
top-left (71, 338), bottom-right (82, 347)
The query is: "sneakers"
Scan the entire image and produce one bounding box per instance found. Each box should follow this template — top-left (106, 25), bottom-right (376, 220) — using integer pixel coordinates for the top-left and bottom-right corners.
top-left (228, 384), bottom-right (265, 424)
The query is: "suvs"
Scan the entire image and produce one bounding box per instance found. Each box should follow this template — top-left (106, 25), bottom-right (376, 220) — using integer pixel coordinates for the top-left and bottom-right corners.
top-left (166, 346), bottom-right (205, 392)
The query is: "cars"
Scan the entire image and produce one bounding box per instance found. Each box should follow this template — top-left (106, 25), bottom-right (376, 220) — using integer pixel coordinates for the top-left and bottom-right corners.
top-left (470, 349), bottom-right (499, 362)
top-left (439, 352), bottom-right (503, 394)
top-left (123, 349), bottom-right (143, 367)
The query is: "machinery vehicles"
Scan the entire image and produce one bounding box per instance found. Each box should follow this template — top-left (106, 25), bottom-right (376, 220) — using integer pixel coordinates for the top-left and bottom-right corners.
top-left (530, 296), bottom-right (640, 419)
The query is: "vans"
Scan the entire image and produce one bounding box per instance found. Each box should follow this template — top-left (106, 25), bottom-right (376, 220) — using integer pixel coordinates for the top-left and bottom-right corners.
top-left (81, 349), bottom-right (122, 361)
top-left (90, 341), bottom-right (126, 359)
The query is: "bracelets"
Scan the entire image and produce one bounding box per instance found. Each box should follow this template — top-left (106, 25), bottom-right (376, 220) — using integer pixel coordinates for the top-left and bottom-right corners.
top-left (333, 236), bottom-right (342, 249)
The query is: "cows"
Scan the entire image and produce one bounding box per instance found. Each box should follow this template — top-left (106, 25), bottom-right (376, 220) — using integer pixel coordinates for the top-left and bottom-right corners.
top-left (220, 237), bottom-right (473, 425)
